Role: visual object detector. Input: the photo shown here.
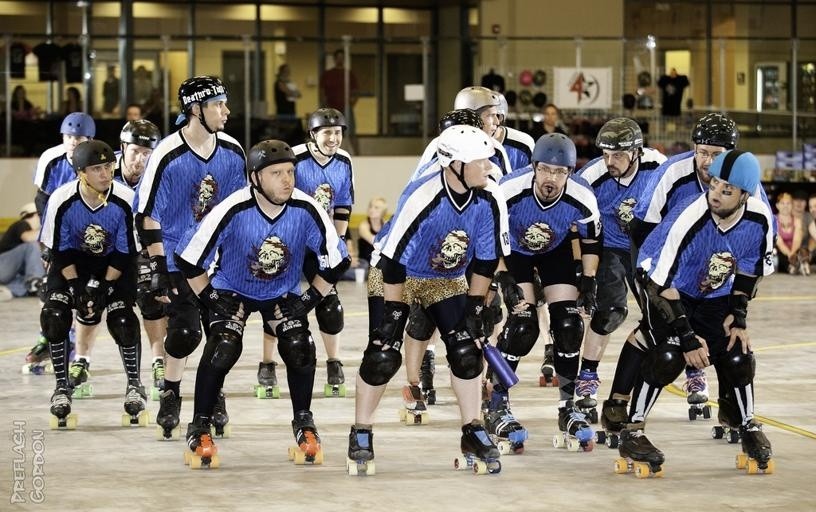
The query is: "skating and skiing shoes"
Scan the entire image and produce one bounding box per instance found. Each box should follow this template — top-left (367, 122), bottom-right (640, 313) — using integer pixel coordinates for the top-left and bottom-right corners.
top-left (151, 359), bottom-right (169, 401)
top-left (64, 357), bottom-right (93, 399)
top-left (20, 341), bottom-right (55, 377)
top-left (210, 392), bottom-right (230, 437)
top-left (453, 423), bottom-right (502, 474)
top-left (595, 399), bottom-right (632, 449)
top-left (684, 371), bottom-right (713, 421)
top-left (399, 386), bottom-right (431, 426)
top-left (26, 277), bottom-right (43, 296)
top-left (480, 378), bottom-right (527, 457)
top-left (421, 386), bottom-right (437, 406)
top-left (539, 351), bottom-right (558, 389)
top-left (552, 401), bottom-right (596, 454)
top-left (736, 426), bottom-right (776, 479)
top-left (121, 376), bottom-right (148, 425)
top-left (613, 426), bottom-right (666, 481)
top-left (155, 388), bottom-right (183, 439)
top-left (255, 360), bottom-right (281, 400)
top-left (574, 371), bottom-right (600, 424)
top-left (323, 358), bottom-right (347, 400)
top-left (712, 417), bottom-right (761, 442)
top-left (347, 423), bottom-right (376, 476)
top-left (289, 413), bottom-right (325, 468)
top-left (183, 413), bottom-right (221, 470)
top-left (49, 382), bottom-right (78, 431)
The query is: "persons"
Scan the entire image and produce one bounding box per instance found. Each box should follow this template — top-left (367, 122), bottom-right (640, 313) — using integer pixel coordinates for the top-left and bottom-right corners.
top-left (2, 64), bottom-right (165, 161)
top-left (575, 116), bottom-right (712, 424)
top-left (274, 64), bottom-right (302, 116)
top-left (254, 106), bottom-right (356, 400)
top-left (347, 126), bottom-right (511, 475)
top-left (25, 112), bottom-right (96, 376)
top-left (613, 148), bottom-right (775, 479)
top-left (136, 74), bottom-right (249, 439)
top-left (121, 106), bottom-right (157, 162)
top-left (529, 104), bottom-right (568, 144)
top-left (38, 140), bottom-right (144, 430)
top-left (496, 132), bottom-right (602, 454)
top-left (771, 189), bottom-right (816, 277)
top-left (174, 139), bottom-right (351, 469)
top-left (61, 120), bottom-right (171, 400)
top-left (568, 119), bottom-right (602, 161)
top-left (595, 111), bottom-right (776, 448)
top-left (321, 50), bottom-right (360, 136)
top-left (481, 89), bottom-right (536, 181)
top-left (400, 84), bottom-right (512, 425)
top-left (357, 195), bottom-right (386, 263)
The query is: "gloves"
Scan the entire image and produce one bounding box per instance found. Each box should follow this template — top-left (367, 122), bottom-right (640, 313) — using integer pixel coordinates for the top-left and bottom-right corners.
top-left (583, 275), bottom-right (603, 313)
top-left (679, 318), bottom-right (702, 354)
top-left (275, 285), bottom-right (324, 320)
top-left (149, 255), bottom-right (180, 294)
top-left (723, 292), bottom-right (749, 328)
top-left (198, 282), bottom-right (245, 322)
top-left (90, 282), bottom-right (114, 319)
top-left (68, 281), bottom-right (91, 323)
top-left (368, 300), bottom-right (410, 352)
top-left (494, 269), bottom-right (525, 307)
top-left (451, 292), bottom-right (487, 340)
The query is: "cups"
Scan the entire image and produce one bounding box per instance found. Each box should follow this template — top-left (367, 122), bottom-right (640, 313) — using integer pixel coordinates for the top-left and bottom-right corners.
top-left (355, 268), bottom-right (365, 284)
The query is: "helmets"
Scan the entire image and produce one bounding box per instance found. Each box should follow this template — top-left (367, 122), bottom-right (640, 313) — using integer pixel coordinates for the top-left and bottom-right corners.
top-left (72, 138), bottom-right (117, 194)
top-left (178, 73), bottom-right (230, 134)
top-left (118, 117), bottom-right (162, 171)
top-left (307, 107), bottom-right (349, 158)
top-left (527, 131), bottom-right (579, 198)
top-left (19, 202), bottom-right (37, 217)
top-left (245, 138), bottom-right (297, 206)
top-left (703, 151), bottom-right (763, 218)
top-left (59, 111), bottom-right (97, 138)
top-left (594, 116), bottom-right (645, 178)
top-left (692, 113), bottom-right (740, 172)
top-left (451, 83), bottom-right (506, 133)
top-left (434, 124), bottom-right (496, 193)
